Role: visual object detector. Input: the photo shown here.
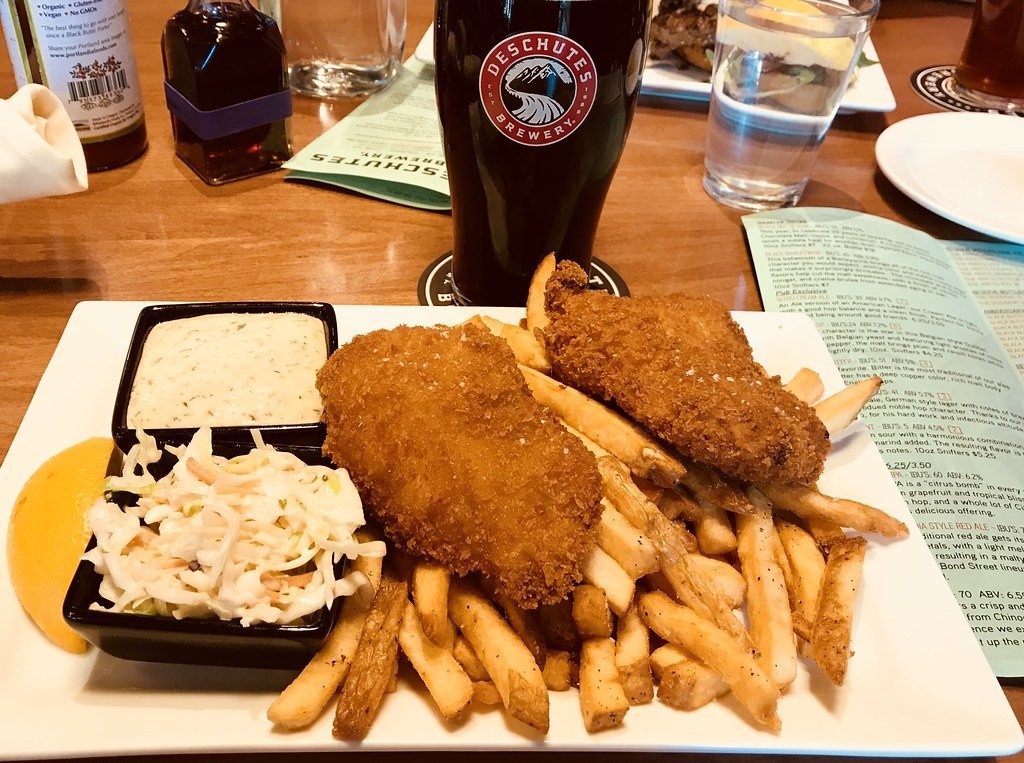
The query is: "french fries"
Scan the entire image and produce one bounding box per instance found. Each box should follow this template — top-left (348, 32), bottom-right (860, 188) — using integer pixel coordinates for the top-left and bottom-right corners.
top-left (264, 251), bottom-right (908, 737)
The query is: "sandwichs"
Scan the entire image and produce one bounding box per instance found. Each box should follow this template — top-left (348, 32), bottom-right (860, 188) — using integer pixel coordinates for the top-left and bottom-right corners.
top-left (647, 0), bottom-right (864, 115)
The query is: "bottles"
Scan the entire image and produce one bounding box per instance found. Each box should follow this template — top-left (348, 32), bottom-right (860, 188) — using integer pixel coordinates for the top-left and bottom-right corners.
top-left (160, 1), bottom-right (293, 186)
top-left (0, 0), bottom-right (148, 177)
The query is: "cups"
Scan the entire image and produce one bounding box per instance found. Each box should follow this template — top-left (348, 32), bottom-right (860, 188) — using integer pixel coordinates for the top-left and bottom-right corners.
top-left (954, 1), bottom-right (1024, 112)
top-left (431, 1), bottom-right (651, 304)
top-left (703, 0), bottom-right (878, 211)
top-left (259, 0), bottom-right (408, 103)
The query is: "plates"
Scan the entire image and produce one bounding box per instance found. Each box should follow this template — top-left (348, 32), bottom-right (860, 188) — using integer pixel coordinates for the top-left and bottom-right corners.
top-left (869, 111), bottom-right (1023, 252)
top-left (111, 299), bottom-right (342, 446)
top-left (60, 447), bottom-right (345, 669)
top-left (0, 295), bottom-right (1024, 762)
top-left (632, 25), bottom-right (895, 116)
